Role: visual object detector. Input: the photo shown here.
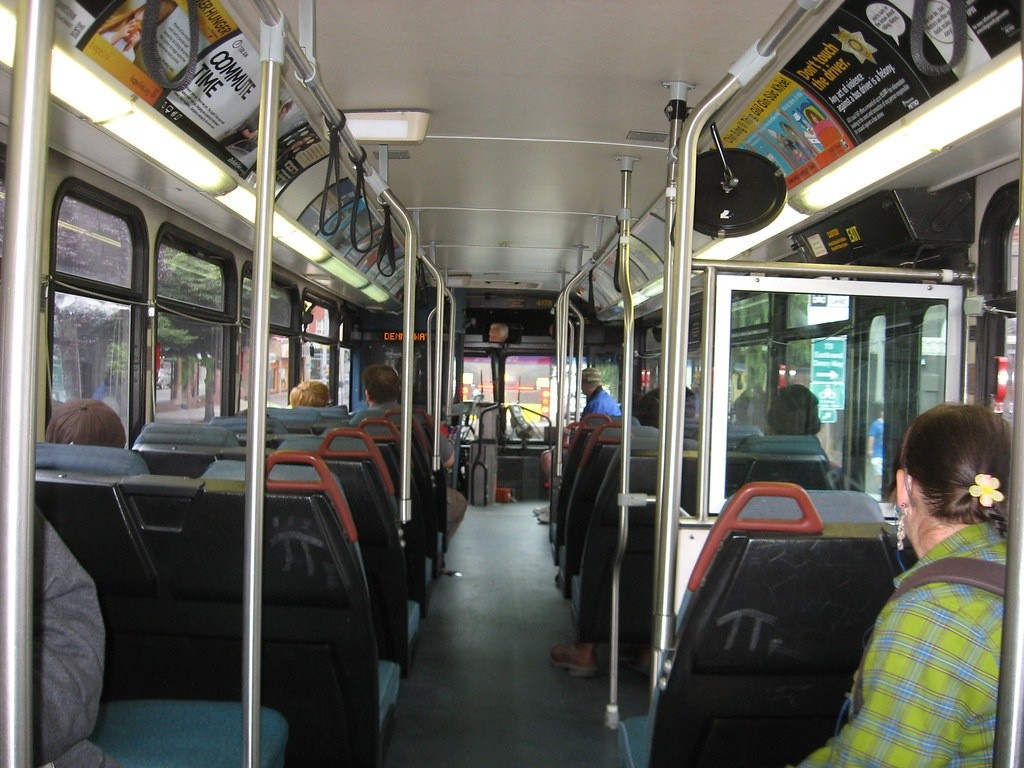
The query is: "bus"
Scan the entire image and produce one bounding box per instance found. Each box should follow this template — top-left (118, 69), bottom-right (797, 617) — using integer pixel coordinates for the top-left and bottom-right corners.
top-left (463, 356), bottom-right (552, 426)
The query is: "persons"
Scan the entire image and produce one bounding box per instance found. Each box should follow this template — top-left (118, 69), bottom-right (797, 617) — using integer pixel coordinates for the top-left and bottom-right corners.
top-left (795, 403), bottom-right (1011, 768)
top-left (350, 366), bottom-right (467, 573)
top-left (100, 0), bottom-right (178, 64)
top-left (534, 368), bottom-right (621, 523)
top-left (766, 384), bottom-right (842, 483)
top-left (33, 504), bottom-right (122, 767)
top-left (277, 134), bottom-right (315, 166)
top-left (636, 387), bottom-right (696, 427)
top-left (869, 408), bottom-right (884, 491)
top-left (47, 399), bottom-right (127, 449)
top-left (290, 381), bottom-right (330, 407)
top-left (489, 323), bottom-right (509, 342)
top-left (223, 102), bottom-right (294, 148)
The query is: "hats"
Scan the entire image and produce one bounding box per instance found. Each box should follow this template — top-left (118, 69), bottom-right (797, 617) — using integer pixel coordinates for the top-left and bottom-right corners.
top-left (581, 368), bottom-right (602, 381)
top-left (45, 399), bottom-right (126, 449)
top-left (766, 385), bottom-right (821, 435)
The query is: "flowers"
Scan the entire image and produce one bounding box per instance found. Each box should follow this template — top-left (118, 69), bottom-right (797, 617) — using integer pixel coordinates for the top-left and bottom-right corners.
top-left (969, 473), bottom-right (1004, 507)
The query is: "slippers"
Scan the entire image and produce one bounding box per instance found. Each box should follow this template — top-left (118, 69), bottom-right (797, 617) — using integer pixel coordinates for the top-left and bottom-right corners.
top-left (551, 643), bottom-right (600, 678)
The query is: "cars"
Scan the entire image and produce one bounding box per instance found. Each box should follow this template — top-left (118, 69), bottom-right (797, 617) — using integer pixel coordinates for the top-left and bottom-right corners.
top-left (156, 368), bottom-right (171, 389)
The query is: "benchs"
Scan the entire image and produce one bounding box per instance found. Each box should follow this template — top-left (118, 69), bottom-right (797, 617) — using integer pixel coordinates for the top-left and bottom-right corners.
top-left (33, 404), bottom-right (447, 768)
top-left (549, 412), bottom-right (920, 768)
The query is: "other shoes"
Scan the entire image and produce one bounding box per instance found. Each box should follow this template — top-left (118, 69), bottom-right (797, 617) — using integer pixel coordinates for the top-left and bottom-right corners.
top-left (532, 506), bottom-right (550, 523)
